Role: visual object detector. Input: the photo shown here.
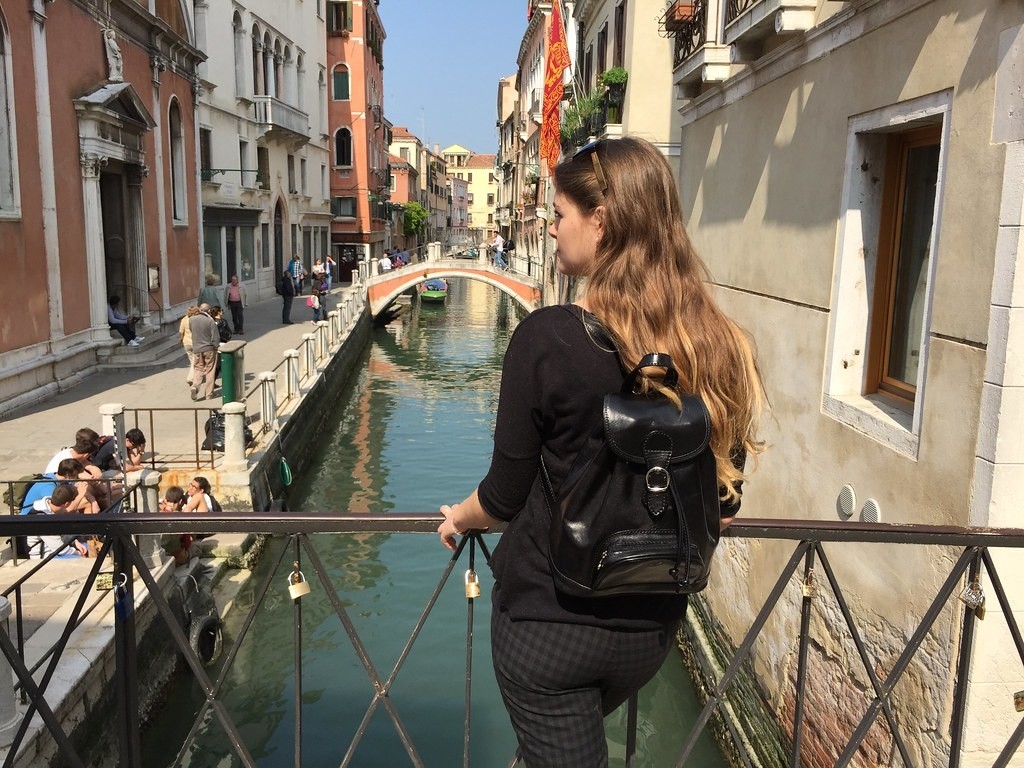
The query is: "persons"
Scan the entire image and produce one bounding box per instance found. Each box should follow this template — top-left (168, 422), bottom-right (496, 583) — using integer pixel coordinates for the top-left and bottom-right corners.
top-left (210, 306), bottom-right (232, 388)
top-left (224, 275), bottom-right (248, 335)
top-left (160, 486), bottom-right (213, 572)
top-left (189, 303), bottom-right (220, 401)
top-left (18, 427), bottom-right (146, 558)
top-left (282, 255), bottom-right (336, 324)
top-left (378, 245), bottom-right (412, 272)
top-left (491, 231), bottom-right (507, 271)
top-left (503, 240), bottom-right (515, 253)
top-left (179, 305), bottom-right (200, 386)
top-left (198, 273), bottom-right (226, 311)
top-left (108, 295), bottom-right (145, 347)
top-left (182, 477), bottom-right (216, 540)
top-left (106, 29), bottom-right (122, 75)
top-left (438, 132), bottom-right (780, 768)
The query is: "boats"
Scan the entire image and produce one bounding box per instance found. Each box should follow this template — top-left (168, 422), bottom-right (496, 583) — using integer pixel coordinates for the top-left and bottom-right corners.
top-left (420, 278), bottom-right (447, 302)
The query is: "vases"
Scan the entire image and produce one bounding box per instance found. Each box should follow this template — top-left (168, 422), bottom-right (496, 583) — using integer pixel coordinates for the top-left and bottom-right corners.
top-left (665, 3), bottom-right (694, 31)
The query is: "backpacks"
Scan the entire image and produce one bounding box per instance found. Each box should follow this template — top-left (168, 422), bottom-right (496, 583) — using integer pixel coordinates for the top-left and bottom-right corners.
top-left (275, 279), bottom-right (283, 295)
top-left (536, 305), bottom-right (720, 598)
top-left (3, 473), bottom-right (57, 509)
top-left (87, 435), bottom-right (113, 461)
top-left (6, 508), bottom-right (46, 559)
top-left (307, 297), bottom-right (312, 307)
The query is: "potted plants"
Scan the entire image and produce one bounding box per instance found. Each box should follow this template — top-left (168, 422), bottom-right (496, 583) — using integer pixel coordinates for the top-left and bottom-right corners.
top-left (557, 66), bottom-right (627, 155)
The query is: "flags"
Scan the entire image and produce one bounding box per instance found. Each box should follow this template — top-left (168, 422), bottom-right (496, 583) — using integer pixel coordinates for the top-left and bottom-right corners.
top-left (540, 0), bottom-right (572, 186)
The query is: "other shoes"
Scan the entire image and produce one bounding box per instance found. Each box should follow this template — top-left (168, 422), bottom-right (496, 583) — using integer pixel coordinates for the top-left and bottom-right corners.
top-left (283, 321), bottom-right (294, 324)
top-left (311, 320), bottom-right (317, 326)
top-left (127, 340), bottom-right (139, 346)
top-left (186, 381), bottom-right (193, 386)
top-left (134, 336), bottom-right (145, 342)
top-left (239, 330), bottom-right (244, 335)
top-left (191, 388), bottom-right (198, 400)
top-left (233, 330), bottom-right (239, 334)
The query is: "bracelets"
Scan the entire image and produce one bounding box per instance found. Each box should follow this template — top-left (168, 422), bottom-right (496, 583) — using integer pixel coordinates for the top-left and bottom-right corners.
top-left (451, 509), bottom-right (470, 536)
top-left (138, 451), bottom-right (145, 455)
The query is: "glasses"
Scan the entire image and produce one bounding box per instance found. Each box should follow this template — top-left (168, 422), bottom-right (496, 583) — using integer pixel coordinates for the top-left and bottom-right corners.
top-left (189, 483), bottom-right (200, 490)
top-left (572, 140), bottom-right (608, 198)
